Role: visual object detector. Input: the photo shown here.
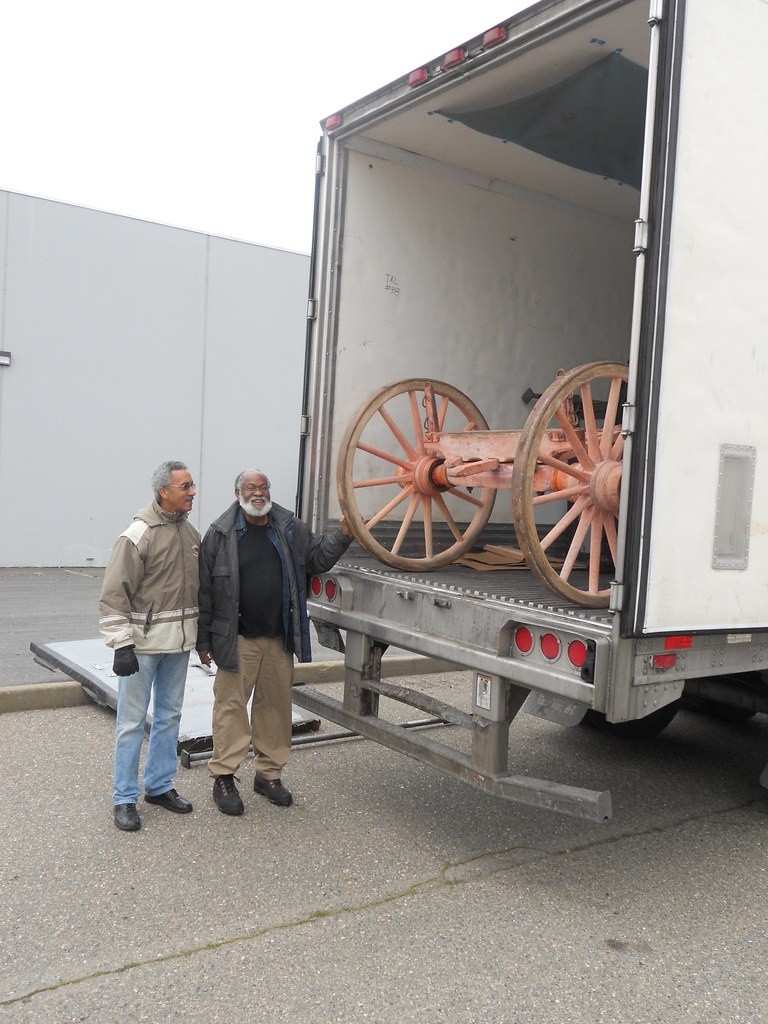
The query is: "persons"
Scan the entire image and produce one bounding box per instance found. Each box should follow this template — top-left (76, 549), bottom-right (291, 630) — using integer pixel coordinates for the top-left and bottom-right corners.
top-left (99, 461), bottom-right (201, 831)
top-left (197, 469), bottom-right (366, 816)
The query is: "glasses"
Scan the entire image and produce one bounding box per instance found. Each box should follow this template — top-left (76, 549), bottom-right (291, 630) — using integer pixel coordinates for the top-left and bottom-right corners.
top-left (168, 484), bottom-right (195, 491)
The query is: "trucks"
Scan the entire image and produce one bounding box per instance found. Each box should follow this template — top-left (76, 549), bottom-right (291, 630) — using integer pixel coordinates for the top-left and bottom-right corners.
top-left (291, 1), bottom-right (768, 822)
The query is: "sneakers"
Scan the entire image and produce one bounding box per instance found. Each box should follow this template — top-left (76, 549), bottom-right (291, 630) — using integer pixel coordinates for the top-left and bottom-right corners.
top-left (113, 803), bottom-right (141, 831)
top-left (145, 787), bottom-right (193, 814)
top-left (254, 774), bottom-right (293, 806)
top-left (213, 775), bottom-right (244, 815)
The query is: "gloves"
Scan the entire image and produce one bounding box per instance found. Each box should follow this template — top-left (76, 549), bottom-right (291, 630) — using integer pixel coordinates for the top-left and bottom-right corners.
top-left (113, 644), bottom-right (140, 677)
